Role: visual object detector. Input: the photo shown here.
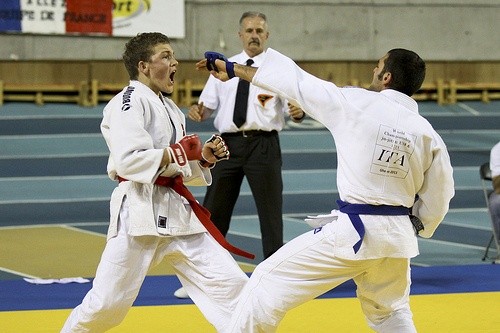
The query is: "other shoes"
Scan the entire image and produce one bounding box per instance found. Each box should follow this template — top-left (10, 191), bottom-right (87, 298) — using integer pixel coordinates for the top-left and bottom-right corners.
top-left (173, 287), bottom-right (190, 299)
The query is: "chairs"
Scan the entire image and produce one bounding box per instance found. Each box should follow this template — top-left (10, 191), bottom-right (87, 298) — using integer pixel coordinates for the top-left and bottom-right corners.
top-left (479, 162), bottom-right (500, 264)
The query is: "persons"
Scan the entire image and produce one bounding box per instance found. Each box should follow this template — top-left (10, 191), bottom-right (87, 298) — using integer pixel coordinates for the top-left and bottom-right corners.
top-left (489, 142), bottom-right (500, 245)
top-left (61, 32), bottom-right (248, 333)
top-left (174, 12), bottom-right (306, 299)
top-left (196, 48), bottom-right (455, 333)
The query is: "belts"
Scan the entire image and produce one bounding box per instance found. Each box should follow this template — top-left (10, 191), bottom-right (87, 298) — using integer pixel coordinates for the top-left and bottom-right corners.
top-left (222, 128), bottom-right (279, 138)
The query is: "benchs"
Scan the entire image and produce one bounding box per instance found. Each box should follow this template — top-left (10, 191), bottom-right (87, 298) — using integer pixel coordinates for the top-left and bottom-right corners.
top-left (0, 79), bottom-right (203, 107)
top-left (410, 79), bottom-right (500, 105)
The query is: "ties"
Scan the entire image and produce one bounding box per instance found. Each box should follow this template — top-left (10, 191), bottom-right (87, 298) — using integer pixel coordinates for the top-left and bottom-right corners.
top-left (232, 59), bottom-right (254, 128)
